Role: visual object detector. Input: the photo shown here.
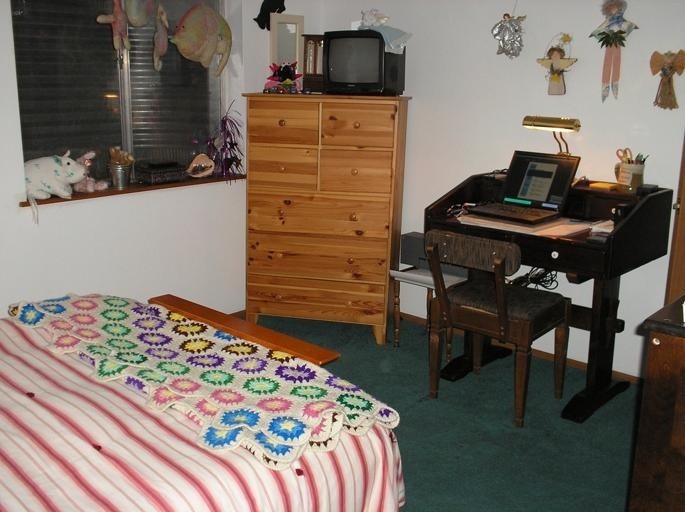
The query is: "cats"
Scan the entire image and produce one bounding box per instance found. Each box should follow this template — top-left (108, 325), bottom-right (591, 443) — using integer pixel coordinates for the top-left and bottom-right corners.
top-left (253, 0), bottom-right (286, 32)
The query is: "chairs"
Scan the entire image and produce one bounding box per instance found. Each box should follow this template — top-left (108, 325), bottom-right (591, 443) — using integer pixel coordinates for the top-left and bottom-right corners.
top-left (422, 231), bottom-right (571, 427)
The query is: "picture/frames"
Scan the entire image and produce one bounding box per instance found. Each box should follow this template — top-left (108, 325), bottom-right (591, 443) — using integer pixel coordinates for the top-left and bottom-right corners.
top-left (270, 15), bottom-right (304, 89)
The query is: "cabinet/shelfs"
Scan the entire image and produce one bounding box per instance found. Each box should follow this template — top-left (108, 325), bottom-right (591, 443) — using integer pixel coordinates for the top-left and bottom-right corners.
top-left (245, 93), bottom-right (411, 346)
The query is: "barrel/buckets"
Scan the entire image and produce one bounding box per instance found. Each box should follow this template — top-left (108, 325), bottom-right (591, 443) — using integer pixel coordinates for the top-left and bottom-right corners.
top-left (108, 164), bottom-right (131, 191)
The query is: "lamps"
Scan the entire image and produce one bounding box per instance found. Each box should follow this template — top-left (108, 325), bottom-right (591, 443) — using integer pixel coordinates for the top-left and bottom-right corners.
top-left (524, 115), bottom-right (581, 157)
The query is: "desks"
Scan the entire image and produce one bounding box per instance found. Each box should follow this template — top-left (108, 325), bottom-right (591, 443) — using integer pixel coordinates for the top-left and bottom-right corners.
top-left (425, 173), bottom-right (673, 424)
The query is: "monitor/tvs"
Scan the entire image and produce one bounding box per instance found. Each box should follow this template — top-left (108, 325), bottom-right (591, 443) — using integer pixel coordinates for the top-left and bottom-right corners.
top-left (323, 30), bottom-right (405, 95)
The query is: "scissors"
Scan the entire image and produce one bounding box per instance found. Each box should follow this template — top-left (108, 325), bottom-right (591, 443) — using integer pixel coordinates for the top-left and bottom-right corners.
top-left (616, 148), bottom-right (632, 163)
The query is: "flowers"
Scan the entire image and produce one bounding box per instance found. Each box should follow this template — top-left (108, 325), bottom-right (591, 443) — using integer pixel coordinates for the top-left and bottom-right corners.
top-left (263, 61), bottom-right (304, 94)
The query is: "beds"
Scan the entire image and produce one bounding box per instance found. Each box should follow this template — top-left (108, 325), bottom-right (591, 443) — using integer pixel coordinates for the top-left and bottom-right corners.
top-left (1, 295), bottom-right (392, 511)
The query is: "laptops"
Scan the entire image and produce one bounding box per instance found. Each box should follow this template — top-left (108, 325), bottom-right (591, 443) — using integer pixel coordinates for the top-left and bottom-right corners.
top-left (467, 150), bottom-right (580, 225)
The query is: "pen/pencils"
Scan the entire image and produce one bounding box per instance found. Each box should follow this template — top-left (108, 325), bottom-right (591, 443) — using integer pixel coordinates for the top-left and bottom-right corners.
top-left (635, 152), bottom-right (650, 163)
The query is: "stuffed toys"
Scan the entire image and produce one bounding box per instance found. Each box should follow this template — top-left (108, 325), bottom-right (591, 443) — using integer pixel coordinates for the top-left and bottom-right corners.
top-left (96, 0), bottom-right (232, 78)
top-left (23, 149), bottom-right (109, 201)
